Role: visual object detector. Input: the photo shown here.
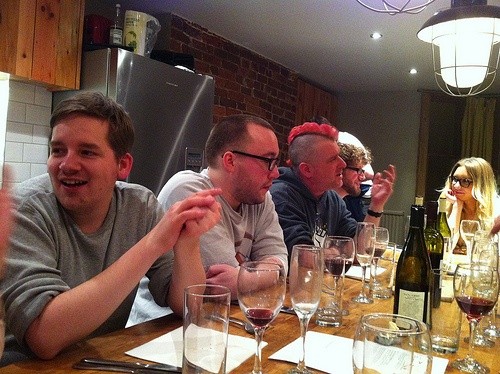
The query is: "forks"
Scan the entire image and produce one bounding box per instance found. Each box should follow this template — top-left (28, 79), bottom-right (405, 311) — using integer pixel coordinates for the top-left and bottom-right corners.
top-left (206, 311), bottom-right (269, 333)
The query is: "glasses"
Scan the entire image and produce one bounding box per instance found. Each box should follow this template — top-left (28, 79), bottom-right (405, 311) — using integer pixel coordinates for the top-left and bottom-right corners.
top-left (343, 166), bottom-right (365, 175)
top-left (449, 176), bottom-right (473, 188)
top-left (222, 150), bottom-right (280, 170)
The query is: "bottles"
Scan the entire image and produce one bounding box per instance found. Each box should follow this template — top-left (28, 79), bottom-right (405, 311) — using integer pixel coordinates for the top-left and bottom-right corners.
top-left (437, 197), bottom-right (451, 270)
top-left (393, 204), bottom-right (433, 336)
top-left (109, 3), bottom-right (124, 46)
top-left (424, 201), bottom-right (444, 309)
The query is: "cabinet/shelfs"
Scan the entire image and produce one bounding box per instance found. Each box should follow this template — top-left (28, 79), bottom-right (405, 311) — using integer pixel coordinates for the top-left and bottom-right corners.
top-left (0, 0), bottom-right (85, 90)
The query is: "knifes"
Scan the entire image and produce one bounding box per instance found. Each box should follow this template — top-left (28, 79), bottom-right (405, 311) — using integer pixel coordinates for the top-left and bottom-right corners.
top-left (231, 299), bottom-right (297, 316)
top-left (73, 357), bottom-right (182, 374)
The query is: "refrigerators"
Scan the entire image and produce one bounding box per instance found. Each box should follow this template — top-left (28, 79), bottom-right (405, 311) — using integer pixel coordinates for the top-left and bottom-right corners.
top-left (51, 48), bottom-right (216, 198)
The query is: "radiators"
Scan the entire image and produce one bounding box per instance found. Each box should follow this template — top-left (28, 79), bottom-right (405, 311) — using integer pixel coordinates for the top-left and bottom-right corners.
top-left (381, 210), bottom-right (404, 246)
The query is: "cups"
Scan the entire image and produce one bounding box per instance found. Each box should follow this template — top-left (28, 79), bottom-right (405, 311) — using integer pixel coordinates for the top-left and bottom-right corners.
top-left (419, 269), bottom-right (466, 355)
top-left (352, 312), bottom-right (433, 374)
top-left (441, 237), bottom-right (452, 273)
top-left (368, 241), bottom-right (396, 299)
top-left (313, 253), bottom-right (347, 328)
top-left (404, 215), bottom-right (428, 246)
top-left (180, 283), bottom-right (232, 374)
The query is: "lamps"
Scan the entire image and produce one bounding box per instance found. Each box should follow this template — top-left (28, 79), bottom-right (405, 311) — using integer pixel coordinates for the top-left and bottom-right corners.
top-left (416, 0), bottom-right (500, 97)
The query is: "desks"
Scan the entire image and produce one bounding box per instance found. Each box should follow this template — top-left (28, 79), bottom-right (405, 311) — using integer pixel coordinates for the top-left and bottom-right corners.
top-left (0, 243), bottom-right (500, 374)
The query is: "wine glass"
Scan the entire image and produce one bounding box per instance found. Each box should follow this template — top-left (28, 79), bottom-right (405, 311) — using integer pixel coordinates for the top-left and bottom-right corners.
top-left (237, 261), bottom-right (286, 374)
top-left (324, 235), bottom-right (356, 316)
top-left (289, 244), bottom-right (324, 374)
top-left (352, 222), bottom-right (390, 304)
top-left (453, 220), bottom-right (500, 374)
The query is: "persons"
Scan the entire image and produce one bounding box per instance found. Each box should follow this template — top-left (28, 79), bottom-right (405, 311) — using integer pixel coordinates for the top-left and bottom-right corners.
top-left (437, 157), bottom-right (500, 255)
top-left (268, 122), bottom-right (395, 269)
top-left (311, 116), bottom-right (375, 205)
top-left (125, 113), bottom-right (289, 332)
top-left (0, 91), bottom-right (220, 374)
top-left (336, 143), bottom-right (370, 223)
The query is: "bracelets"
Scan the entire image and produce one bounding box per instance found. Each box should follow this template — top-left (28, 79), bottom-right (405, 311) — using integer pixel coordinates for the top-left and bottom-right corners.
top-left (367, 208), bottom-right (383, 218)
